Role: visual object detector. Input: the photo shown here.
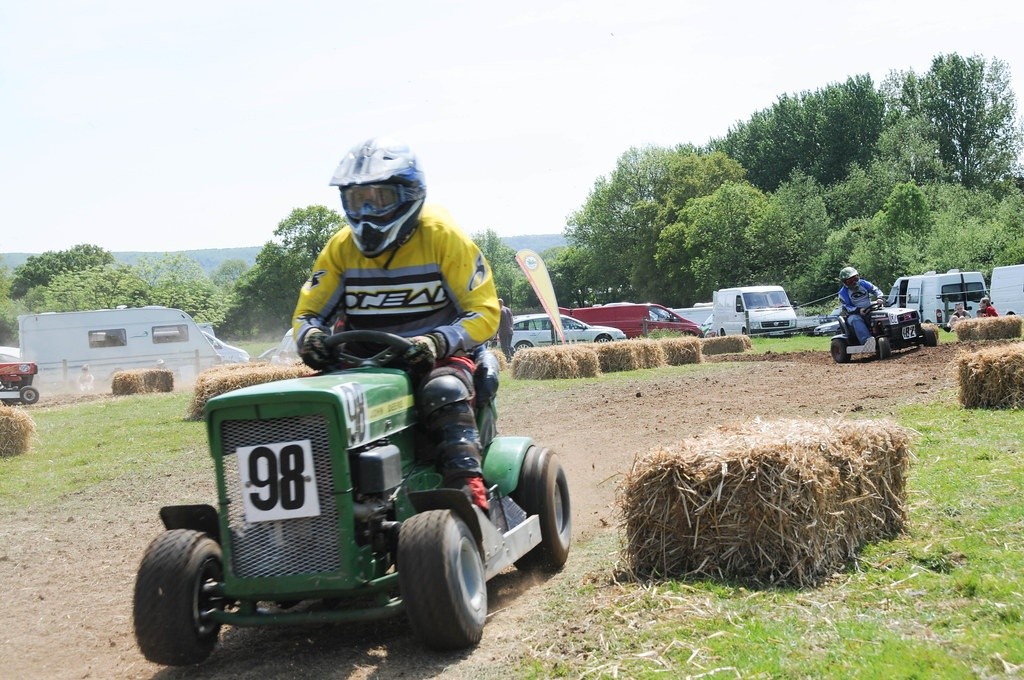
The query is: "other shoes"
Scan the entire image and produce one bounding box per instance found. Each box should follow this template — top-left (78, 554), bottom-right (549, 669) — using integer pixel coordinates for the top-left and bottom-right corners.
top-left (464, 478), bottom-right (490, 514)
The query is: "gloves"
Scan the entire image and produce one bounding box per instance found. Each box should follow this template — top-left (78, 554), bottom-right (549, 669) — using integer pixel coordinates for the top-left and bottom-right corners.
top-left (399, 333), bottom-right (442, 376)
top-left (302, 328), bottom-right (337, 365)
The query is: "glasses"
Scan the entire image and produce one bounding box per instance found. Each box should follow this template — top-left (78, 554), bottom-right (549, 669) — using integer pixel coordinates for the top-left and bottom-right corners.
top-left (847, 276), bottom-right (860, 284)
top-left (340, 183), bottom-right (404, 217)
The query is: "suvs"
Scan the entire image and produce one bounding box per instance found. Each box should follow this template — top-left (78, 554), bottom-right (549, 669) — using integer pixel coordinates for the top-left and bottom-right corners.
top-left (557, 303), bottom-right (704, 340)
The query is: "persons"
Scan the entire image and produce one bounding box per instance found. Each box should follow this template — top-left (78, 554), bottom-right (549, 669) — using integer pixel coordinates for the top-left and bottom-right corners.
top-left (838, 267), bottom-right (883, 346)
top-left (291, 136), bottom-right (500, 521)
top-left (947, 302), bottom-right (972, 333)
top-left (497, 299), bottom-right (515, 363)
top-left (977, 297), bottom-right (998, 318)
top-left (78, 364), bottom-right (94, 394)
top-left (156, 360), bottom-right (166, 369)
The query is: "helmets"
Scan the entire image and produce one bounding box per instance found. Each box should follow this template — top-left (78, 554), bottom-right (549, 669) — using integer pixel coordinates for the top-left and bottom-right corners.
top-left (839, 266), bottom-right (860, 289)
top-left (328, 135), bottom-right (427, 259)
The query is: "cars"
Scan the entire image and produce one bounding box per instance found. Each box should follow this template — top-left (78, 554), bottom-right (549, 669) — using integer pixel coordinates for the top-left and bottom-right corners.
top-left (256, 347), bottom-right (278, 365)
top-left (494, 312), bottom-right (628, 355)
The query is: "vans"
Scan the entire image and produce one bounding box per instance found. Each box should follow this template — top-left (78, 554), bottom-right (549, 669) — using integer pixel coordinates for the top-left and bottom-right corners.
top-left (152, 330), bottom-right (250, 364)
top-left (988, 263), bottom-right (1024, 317)
top-left (884, 268), bottom-right (988, 333)
top-left (15, 306), bottom-right (224, 390)
top-left (713, 285), bottom-right (798, 338)
top-left (270, 326), bottom-right (301, 366)
